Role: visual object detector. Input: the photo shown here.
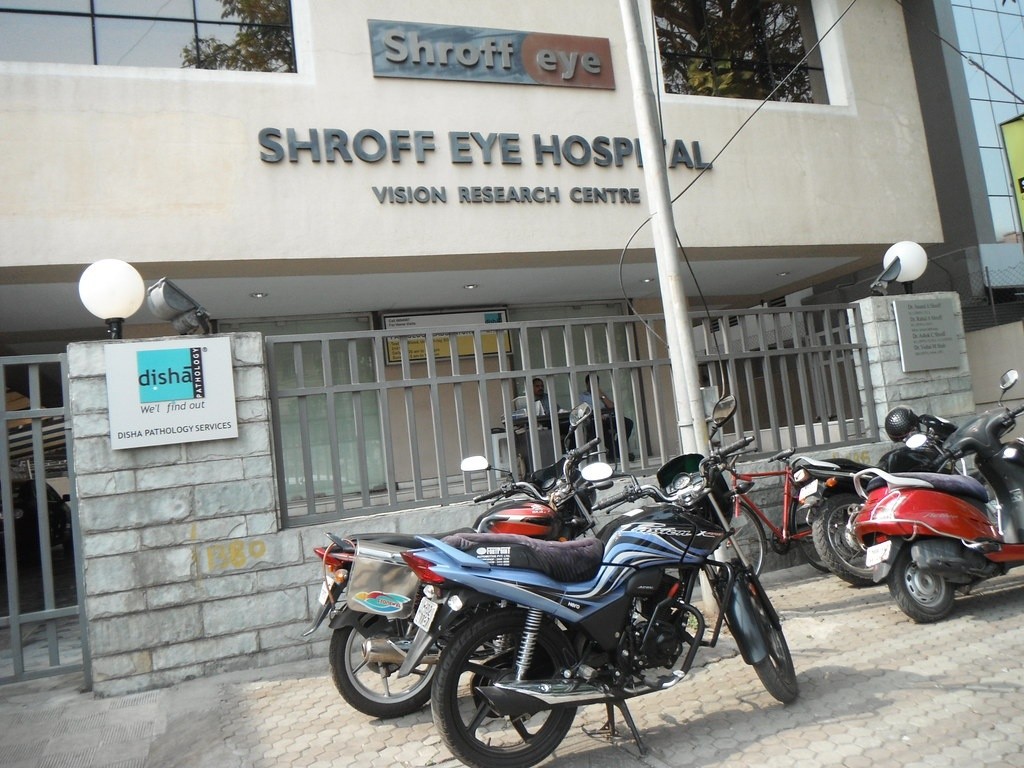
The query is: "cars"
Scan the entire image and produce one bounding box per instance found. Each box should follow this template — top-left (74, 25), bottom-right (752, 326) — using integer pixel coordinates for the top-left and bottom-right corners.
top-left (0, 477), bottom-right (72, 570)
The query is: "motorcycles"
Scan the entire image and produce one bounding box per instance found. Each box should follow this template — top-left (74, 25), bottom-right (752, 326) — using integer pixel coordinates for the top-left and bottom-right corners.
top-left (396, 396), bottom-right (807, 768)
top-left (792, 406), bottom-right (999, 588)
top-left (299, 401), bottom-right (616, 721)
top-left (850, 368), bottom-right (1024, 623)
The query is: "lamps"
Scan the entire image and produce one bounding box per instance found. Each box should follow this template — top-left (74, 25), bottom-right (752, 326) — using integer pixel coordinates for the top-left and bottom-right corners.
top-left (78, 258), bottom-right (146, 338)
top-left (882, 240), bottom-right (928, 294)
top-left (145, 277), bottom-right (212, 335)
top-left (868, 256), bottom-right (901, 296)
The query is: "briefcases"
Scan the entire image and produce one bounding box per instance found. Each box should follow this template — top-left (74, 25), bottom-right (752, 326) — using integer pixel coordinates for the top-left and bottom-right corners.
top-left (347, 540), bottom-right (421, 619)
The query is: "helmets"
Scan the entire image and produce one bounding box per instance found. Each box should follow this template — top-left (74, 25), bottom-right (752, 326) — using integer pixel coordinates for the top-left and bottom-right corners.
top-left (886, 406), bottom-right (920, 442)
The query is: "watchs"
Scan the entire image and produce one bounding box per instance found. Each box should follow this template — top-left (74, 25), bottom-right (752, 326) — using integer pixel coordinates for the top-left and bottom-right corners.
top-left (601, 395), bottom-right (607, 401)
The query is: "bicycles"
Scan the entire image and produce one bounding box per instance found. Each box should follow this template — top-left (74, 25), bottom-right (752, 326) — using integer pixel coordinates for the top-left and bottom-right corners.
top-left (712, 445), bottom-right (831, 573)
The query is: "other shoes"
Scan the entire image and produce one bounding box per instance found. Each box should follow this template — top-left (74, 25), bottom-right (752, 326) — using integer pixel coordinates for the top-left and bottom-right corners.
top-left (617, 452), bottom-right (635, 462)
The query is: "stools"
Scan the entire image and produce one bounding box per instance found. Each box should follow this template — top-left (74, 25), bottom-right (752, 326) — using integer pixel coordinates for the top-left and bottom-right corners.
top-left (491, 432), bottom-right (521, 478)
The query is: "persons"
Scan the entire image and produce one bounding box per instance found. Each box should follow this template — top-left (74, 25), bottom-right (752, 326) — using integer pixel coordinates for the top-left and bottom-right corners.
top-left (533, 378), bottom-right (576, 450)
top-left (579, 374), bottom-right (635, 461)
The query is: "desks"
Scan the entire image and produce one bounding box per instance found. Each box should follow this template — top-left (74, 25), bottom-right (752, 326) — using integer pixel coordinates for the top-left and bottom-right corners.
top-left (503, 407), bottom-right (616, 477)
